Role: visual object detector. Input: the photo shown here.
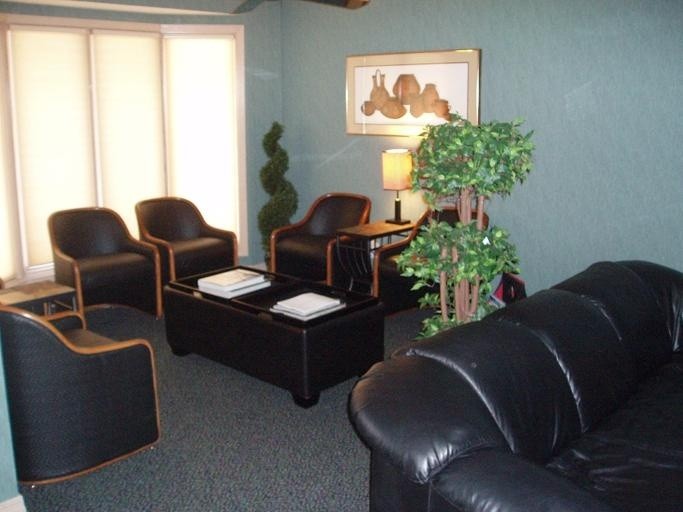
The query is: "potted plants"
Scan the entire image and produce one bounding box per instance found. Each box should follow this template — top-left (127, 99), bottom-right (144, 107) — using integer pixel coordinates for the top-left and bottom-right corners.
top-left (390, 118), bottom-right (536, 359)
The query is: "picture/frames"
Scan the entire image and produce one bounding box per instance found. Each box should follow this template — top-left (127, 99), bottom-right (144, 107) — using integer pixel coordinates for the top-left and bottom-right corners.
top-left (345, 48), bottom-right (480, 138)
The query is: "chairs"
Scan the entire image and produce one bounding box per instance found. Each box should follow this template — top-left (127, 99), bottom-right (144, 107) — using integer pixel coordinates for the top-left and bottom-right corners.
top-left (47, 205), bottom-right (161, 321)
top-left (1, 303), bottom-right (163, 487)
top-left (374, 205), bottom-right (490, 318)
top-left (134, 197), bottom-right (240, 283)
top-left (268, 194), bottom-right (373, 298)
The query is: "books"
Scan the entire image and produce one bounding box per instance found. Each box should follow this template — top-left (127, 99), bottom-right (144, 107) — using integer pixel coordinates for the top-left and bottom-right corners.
top-left (196, 266), bottom-right (266, 293)
top-left (268, 290), bottom-right (349, 322)
top-left (198, 279), bottom-right (272, 299)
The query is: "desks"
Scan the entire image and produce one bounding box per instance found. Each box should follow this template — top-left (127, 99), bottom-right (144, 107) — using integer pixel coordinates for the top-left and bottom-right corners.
top-left (330, 214), bottom-right (409, 297)
top-left (164, 265), bottom-right (384, 406)
top-left (0, 275), bottom-right (76, 313)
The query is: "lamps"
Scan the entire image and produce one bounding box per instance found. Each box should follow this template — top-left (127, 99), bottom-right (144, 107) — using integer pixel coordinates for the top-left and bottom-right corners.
top-left (381, 149), bottom-right (415, 225)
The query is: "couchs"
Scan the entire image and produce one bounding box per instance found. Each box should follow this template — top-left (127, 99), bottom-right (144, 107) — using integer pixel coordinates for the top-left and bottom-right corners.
top-left (348, 256), bottom-right (683, 512)
